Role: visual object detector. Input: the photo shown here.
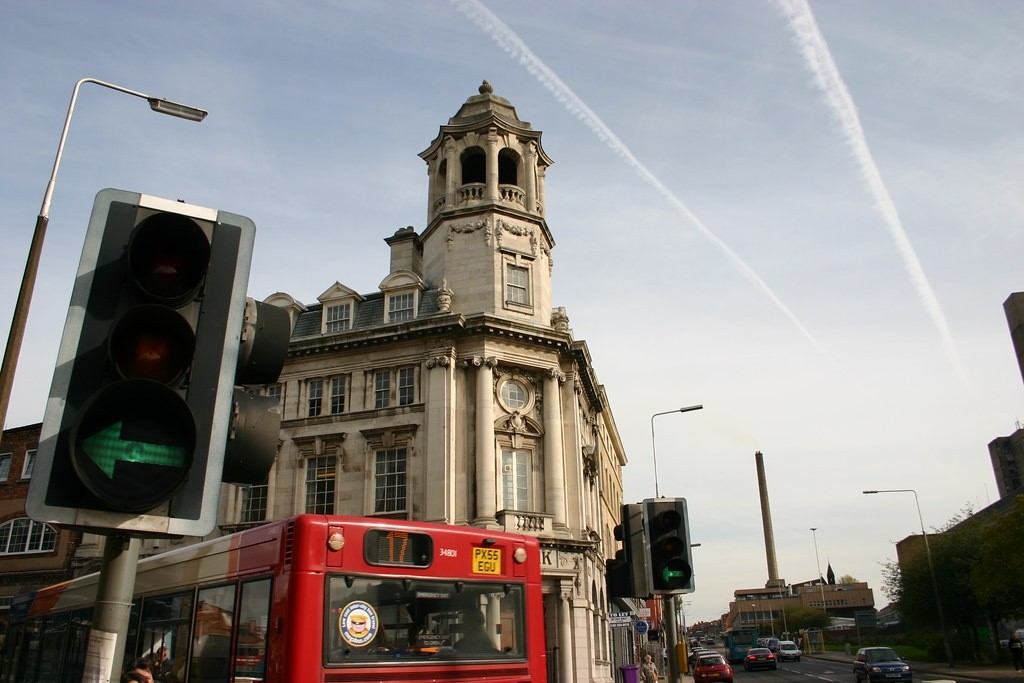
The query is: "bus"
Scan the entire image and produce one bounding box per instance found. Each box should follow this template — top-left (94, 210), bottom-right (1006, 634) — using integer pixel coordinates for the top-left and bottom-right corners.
top-left (0, 512), bottom-right (547, 683)
top-left (720, 628), bottom-right (759, 663)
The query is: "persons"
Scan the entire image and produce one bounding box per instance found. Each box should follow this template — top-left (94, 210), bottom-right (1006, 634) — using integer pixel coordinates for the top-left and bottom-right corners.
top-left (640, 654), bottom-right (658, 683)
top-left (329, 598), bottom-right (390, 652)
top-left (453, 610), bottom-right (493, 653)
top-left (124, 646), bottom-right (173, 683)
top-left (249, 619), bottom-right (257, 635)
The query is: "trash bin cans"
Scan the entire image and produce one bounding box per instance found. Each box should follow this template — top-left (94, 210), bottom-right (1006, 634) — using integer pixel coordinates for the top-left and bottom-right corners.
top-left (619, 664), bottom-right (640, 683)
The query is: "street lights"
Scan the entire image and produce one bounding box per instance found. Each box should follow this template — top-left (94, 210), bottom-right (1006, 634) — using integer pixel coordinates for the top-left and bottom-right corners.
top-left (0, 77), bottom-right (209, 439)
top-left (651, 404), bottom-right (704, 497)
top-left (862, 490), bottom-right (955, 668)
top-left (810, 528), bottom-right (826, 615)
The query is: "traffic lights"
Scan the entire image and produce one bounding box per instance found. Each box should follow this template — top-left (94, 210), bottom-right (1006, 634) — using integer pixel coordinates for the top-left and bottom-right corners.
top-left (221, 295), bottom-right (294, 488)
top-left (643, 497), bottom-right (696, 593)
top-left (24, 188), bottom-right (259, 538)
top-left (613, 503), bottom-right (651, 600)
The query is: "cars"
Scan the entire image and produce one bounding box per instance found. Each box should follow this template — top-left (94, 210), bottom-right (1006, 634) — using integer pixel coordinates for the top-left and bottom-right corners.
top-left (758, 637), bottom-right (779, 650)
top-left (686, 635), bottom-right (734, 683)
top-left (743, 648), bottom-right (776, 671)
top-left (776, 640), bottom-right (801, 663)
top-left (853, 647), bottom-right (913, 683)
